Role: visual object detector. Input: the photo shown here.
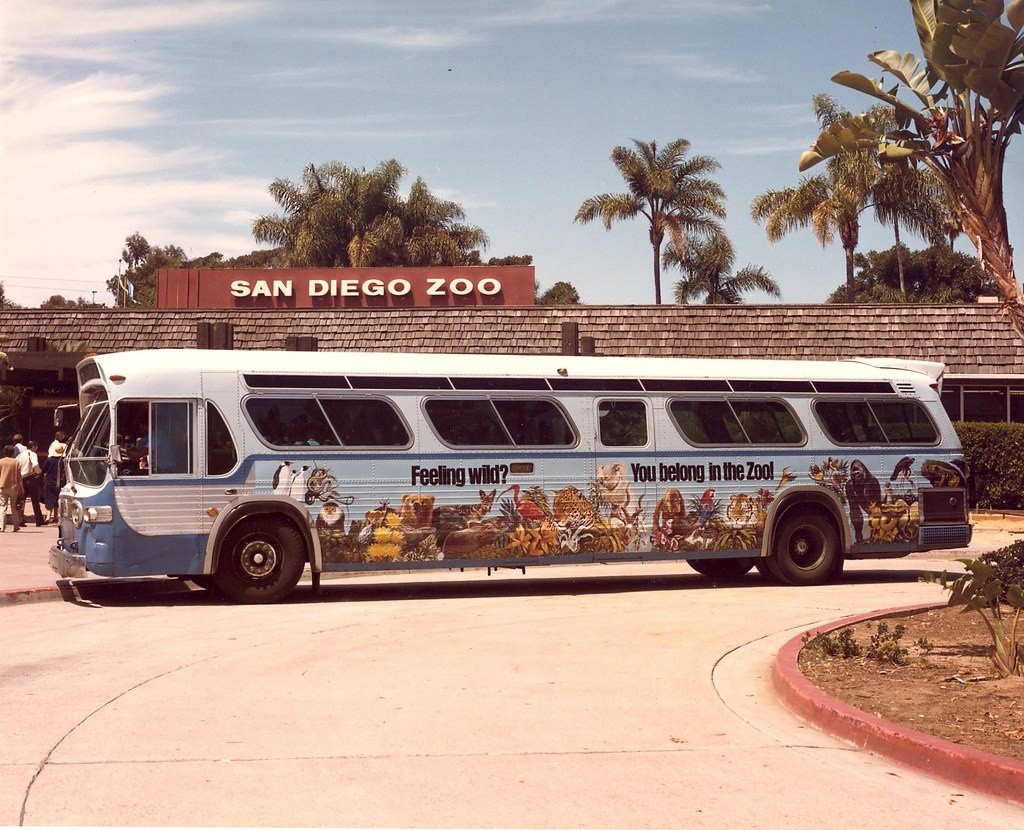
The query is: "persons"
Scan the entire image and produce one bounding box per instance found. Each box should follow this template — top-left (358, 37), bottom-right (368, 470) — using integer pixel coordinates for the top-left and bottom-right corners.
top-left (118, 424), bottom-right (148, 475)
top-left (0, 431), bottom-right (68, 533)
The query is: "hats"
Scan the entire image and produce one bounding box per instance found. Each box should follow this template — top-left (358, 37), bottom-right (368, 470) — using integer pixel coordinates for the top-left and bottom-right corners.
top-left (50, 444), bottom-right (66, 457)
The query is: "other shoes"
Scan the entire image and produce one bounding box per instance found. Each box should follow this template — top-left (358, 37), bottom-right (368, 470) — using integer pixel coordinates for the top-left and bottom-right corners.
top-left (12, 526), bottom-right (20, 532)
top-left (36, 520), bottom-right (49, 527)
top-left (19, 524), bottom-right (28, 527)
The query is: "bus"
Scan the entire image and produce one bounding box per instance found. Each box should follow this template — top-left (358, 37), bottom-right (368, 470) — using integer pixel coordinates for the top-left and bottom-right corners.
top-left (46, 348), bottom-right (975, 605)
